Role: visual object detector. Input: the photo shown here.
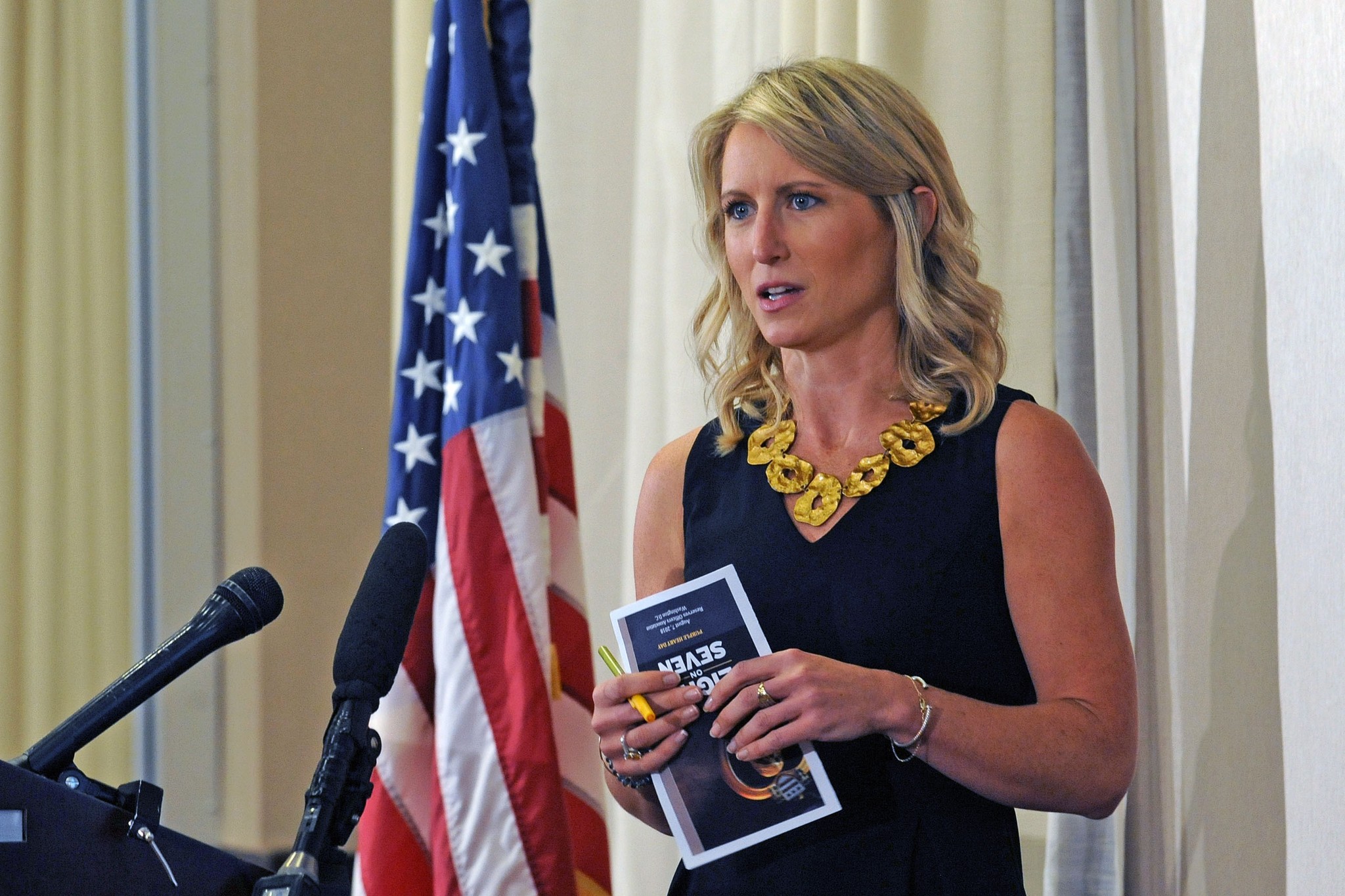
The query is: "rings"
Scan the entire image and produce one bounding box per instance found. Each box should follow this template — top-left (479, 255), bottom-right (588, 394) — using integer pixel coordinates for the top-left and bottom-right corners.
top-left (620, 728), bottom-right (643, 761)
top-left (758, 681), bottom-right (776, 707)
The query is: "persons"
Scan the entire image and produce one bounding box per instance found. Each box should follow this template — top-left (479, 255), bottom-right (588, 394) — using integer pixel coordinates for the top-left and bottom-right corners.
top-left (591, 61), bottom-right (1136, 896)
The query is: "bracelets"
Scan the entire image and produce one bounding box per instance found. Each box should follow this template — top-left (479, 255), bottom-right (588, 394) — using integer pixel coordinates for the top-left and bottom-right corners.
top-left (893, 676), bottom-right (932, 747)
top-left (891, 674), bottom-right (926, 762)
top-left (605, 757), bottom-right (650, 788)
top-left (598, 736), bottom-right (615, 777)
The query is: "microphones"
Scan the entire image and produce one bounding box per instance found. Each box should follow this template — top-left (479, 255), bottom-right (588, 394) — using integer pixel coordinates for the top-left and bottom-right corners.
top-left (253, 522), bottom-right (433, 896)
top-left (15, 564), bottom-right (284, 780)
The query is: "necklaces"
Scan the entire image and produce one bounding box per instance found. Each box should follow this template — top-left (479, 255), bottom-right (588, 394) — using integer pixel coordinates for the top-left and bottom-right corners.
top-left (747, 389), bottom-right (950, 525)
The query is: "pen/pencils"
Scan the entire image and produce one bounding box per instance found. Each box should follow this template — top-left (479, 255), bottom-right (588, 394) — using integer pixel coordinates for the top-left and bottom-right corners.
top-left (598, 645), bottom-right (655, 724)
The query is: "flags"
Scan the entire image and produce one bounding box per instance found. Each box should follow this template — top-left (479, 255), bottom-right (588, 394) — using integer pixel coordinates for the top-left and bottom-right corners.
top-left (352, 0), bottom-right (613, 896)
